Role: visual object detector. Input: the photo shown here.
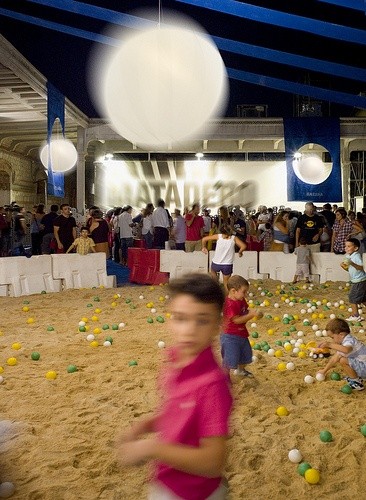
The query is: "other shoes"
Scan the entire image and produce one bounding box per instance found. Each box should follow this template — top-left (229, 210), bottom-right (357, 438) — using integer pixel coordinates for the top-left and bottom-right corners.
top-left (292, 282), bottom-right (298, 284)
top-left (359, 317), bottom-right (365, 321)
top-left (347, 379), bottom-right (364, 391)
top-left (343, 377), bottom-right (363, 383)
top-left (344, 314), bottom-right (361, 322)
top-left (306, 281), bottom-right (311, 284)
top-left (347, 307), bottom-right (363, 314)
top-left (233, 368), bottom-right (254, 378)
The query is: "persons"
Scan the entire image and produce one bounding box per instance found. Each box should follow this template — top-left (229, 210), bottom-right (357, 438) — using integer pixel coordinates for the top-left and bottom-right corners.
top-left (118, 273), bottom-right (234, 500)
top-left (341, 238), bottom-right (366, 321)
top-left (318, 318), bottom-right (366, 390)
top-left (66, 227), bottom-right (96, 254)
top-left (293, 239), bottom-right (313, 284)
top-left (202, 224), bottom-right (247, 292)
top-left (220, 274), bottom-right (256, 377)
top-left (0, 199), bottom-right (366, 265)
